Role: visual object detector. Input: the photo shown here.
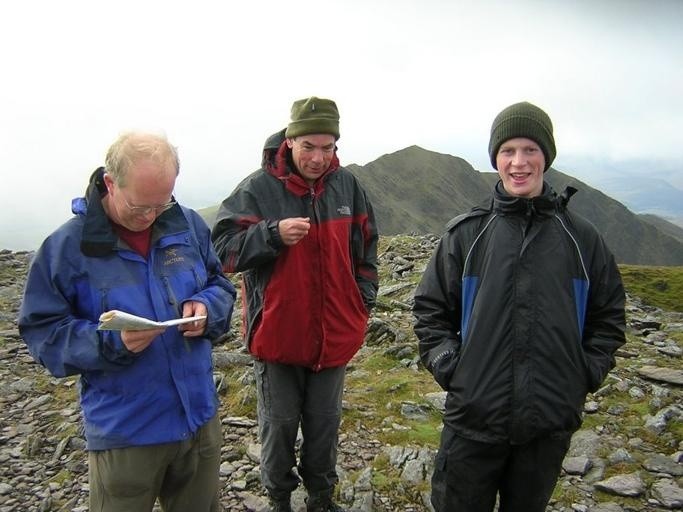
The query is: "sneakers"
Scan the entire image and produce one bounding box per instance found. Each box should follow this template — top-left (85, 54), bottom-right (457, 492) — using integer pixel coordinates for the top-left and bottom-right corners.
top-left (269, 491), bottom-right (293, 512)
top-left (305, 487), bottom-right (350, 512)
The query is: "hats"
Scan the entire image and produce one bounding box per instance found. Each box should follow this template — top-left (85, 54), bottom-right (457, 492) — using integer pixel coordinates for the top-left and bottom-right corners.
top-left (487, 100), bottom-right (557, 174)
top-left (284, 96), bottom-right (340, 141)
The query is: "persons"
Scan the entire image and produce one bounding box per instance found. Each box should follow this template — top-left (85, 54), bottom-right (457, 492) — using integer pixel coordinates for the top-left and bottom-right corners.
top-left (210, 95), bottom-right (379, 511)
top-left (410, 102), bottom-right (627, 511)
top-left (18, 131), bottom-right (236, 511)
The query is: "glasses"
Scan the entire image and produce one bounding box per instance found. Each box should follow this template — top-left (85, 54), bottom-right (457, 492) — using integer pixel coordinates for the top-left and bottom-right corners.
top-left (115, 179), bottom-right (179, 215)
